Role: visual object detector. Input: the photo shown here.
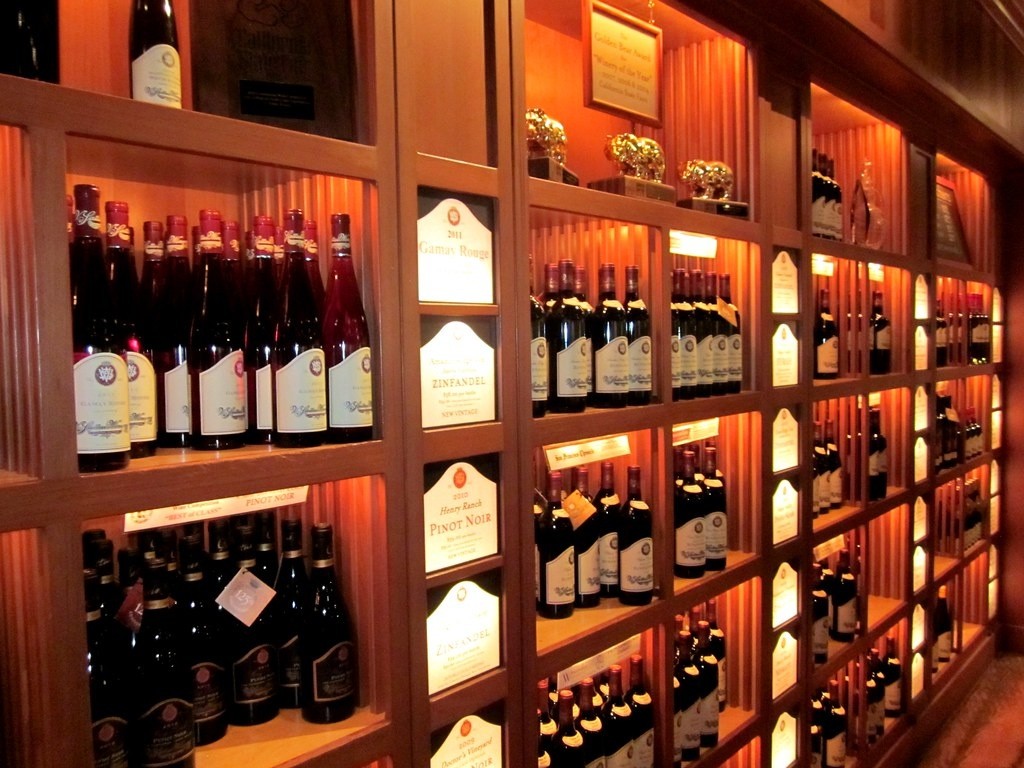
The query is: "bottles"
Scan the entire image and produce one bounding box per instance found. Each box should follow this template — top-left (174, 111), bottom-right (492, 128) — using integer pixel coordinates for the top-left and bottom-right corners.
top-left (536, 654), bottom-right (656, 768)
top-left (811, 549), bottom-right (858, 661)
top-left (675, 438), bottom-right (728, 578)
top-left (82, 513), bottom-right (359, 768)
top-left (59, 184), bottom-right (375, 470)
top-left (848, 637), bottom-right (903, 747)
top-left (958, 294), bottom-right (989, 362)
top-left (528, 254), bottom-right (651, 419)
top-left (128, 0), bottom-right (180, 108)
top-left (673, 597), bottom-right (728, 768)
top-left (814, 289), bottom-right (840, 379)
top-left (811, 149), bottom-right (842, 238)
top-left (857, 409), bottom-right (888, 501)
top-left (533, 463), bottom-right (655, 619)
top-left (936, 300), bottom-right (954, 365)
top-left (941, 478), bottom-right (985, 552)
top-left (669, 268), bottom-right (742, 400)
top-left (813, 420), bottom-right (843, 515)
top-left (964, 404), bottom-right (985, 459)
top-left (811, 680), bottom-right (846, 768)
top-left (934, 395), bottom-right (957, 469)
top-left (845, 291), bottom-right (890, 374)
top-left (930, 586), bottom-right (953, 671)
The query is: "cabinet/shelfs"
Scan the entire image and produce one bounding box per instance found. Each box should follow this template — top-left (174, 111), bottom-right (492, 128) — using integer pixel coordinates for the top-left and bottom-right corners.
top-left (0, 0), bottom-right (1024, 768)
